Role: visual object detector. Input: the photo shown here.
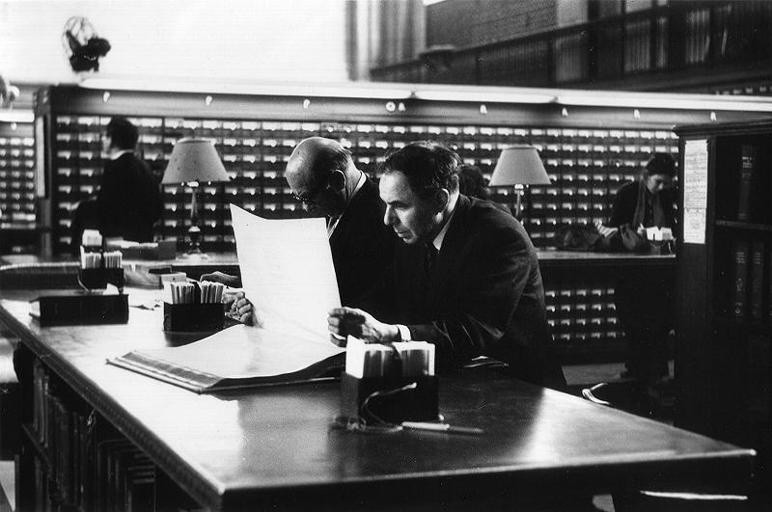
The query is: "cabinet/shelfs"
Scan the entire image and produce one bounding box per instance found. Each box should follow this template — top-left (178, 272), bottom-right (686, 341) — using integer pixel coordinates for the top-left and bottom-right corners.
top-left (670, 116), bottom-right (772, 511)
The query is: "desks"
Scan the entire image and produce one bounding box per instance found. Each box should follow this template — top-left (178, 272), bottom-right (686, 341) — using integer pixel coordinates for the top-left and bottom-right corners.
top-left (1, 286), bottom-right (756, 512)
top-left (0, 250), bottom-right (678, 273)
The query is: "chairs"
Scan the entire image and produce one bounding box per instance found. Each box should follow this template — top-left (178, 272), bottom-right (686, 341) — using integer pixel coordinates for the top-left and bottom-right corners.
top-left (582, 379), bottom-right (749, 511)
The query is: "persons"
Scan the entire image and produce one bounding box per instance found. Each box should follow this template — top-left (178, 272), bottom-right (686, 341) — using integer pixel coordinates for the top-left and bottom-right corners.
top-left (70, 118), bottom-right (165, 243)
top-left (456, 163), bottom-right (512, 215)
top-left (227, 139), bottom-right (569, 394)
top-left (198, 135), bottom-right (402, 323)
top-left (610, 152), bottom-right (677, 380)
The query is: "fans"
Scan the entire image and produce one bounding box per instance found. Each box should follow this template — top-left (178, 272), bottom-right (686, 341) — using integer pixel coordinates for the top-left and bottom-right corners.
top-left (60, 16), bottom-right (110, 73)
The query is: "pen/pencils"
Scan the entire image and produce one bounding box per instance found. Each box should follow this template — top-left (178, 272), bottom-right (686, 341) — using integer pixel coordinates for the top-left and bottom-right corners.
top-left (402, 421), bottom-right (484, 434)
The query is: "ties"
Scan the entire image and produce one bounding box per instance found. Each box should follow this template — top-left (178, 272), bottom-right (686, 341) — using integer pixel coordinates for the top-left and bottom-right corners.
top-left (423, 242), bottom-right (439, 275)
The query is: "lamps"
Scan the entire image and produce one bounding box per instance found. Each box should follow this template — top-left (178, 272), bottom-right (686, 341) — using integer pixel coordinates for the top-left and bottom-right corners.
top-left (159, 138), bottom-right (230, 253)
top-left (487, 145), bottom-right (551, 222)
top-left (385, 100), bottom-right (417, 112)
